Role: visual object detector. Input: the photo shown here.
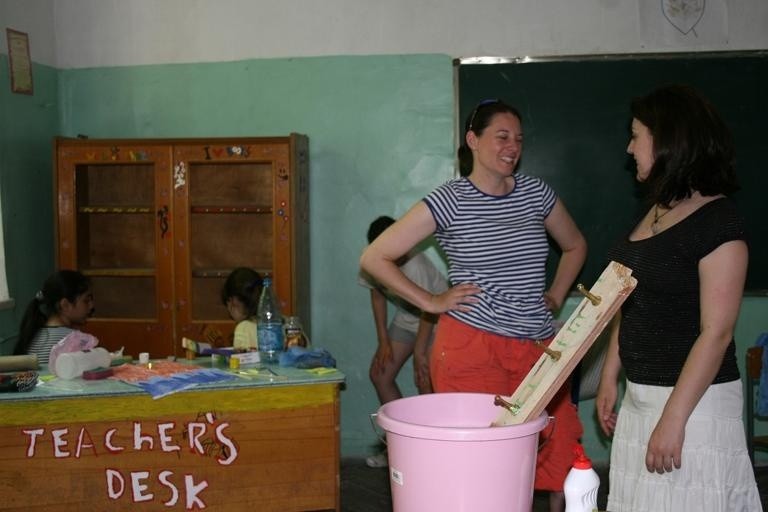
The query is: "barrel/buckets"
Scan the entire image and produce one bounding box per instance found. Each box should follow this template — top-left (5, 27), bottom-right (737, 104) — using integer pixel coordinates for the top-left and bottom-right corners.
top-left (369, 392), bottom-right (554, 512)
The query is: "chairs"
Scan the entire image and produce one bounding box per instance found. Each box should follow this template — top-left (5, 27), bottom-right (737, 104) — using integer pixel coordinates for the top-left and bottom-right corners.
top-left (745, 346), bottom-right (768, 468)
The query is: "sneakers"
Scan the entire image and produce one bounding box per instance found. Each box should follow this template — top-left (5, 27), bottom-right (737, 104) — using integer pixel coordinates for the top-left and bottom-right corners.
top-left (366, 454), bottom-right (388, 468)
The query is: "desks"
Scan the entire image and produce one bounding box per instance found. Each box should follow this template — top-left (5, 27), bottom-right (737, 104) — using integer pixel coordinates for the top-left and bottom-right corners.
top-left (0, 357), bottom-right (347, 512)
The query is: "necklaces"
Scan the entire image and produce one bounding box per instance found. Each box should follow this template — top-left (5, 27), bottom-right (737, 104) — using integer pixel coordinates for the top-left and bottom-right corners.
top-left (650, 199), bottom-right (684, 234)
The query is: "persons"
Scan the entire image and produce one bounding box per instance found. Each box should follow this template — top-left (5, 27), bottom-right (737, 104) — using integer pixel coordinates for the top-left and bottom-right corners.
top-left (357, 216), bottom-right (451, 454)
top-left (12, 269), bottom-right (96, 377)
top-left (352, 97), bottom-right (583, 512)
top-left (221, 266), bottom-right (307, 357)
top-left (594, 89), bottom-right (762, 512)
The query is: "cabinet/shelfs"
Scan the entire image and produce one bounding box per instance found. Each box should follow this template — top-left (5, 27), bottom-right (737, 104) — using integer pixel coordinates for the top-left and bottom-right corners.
top-left (52, 132), bottom-right (311, 359)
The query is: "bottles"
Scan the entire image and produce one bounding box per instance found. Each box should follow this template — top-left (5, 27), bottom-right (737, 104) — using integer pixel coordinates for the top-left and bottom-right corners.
top-left (283, 316), bottom-right (302, 337)
top-left (55, 346), bottom-right (111, 381)
top-left (256, 276), bottom-right (283, 363)
